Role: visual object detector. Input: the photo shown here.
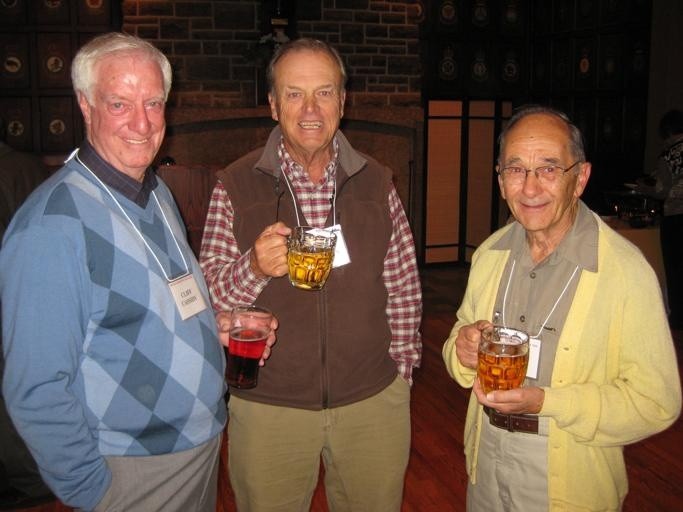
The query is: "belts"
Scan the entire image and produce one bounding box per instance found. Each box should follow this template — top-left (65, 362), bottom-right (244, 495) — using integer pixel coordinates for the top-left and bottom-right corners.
top-left (482, 404), bottom-right (539, 435)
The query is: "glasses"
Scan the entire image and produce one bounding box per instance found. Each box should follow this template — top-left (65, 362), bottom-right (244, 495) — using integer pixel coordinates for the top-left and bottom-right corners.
top-left (496, 160), bottom-right (581, 182)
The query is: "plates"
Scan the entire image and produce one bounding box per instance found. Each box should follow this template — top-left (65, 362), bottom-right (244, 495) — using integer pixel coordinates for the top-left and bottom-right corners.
top-left (624, 183), bottom-right (639, 190)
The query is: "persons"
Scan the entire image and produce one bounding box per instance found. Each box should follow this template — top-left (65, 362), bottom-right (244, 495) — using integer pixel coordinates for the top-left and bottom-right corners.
top-left (442, 107), bottom-right (682, 512)
top-left (0, 32), bottom-right (279, 512)
top-left (635, 112), bottom-right (683, 329)
top-left (199, 37), bottom-right (424, 511)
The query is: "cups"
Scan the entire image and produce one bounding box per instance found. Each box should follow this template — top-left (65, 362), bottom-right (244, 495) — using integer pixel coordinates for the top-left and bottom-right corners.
top-left (225, 305), bottom-right (273, 390)
top-left (286, 226), bottom-right (337, 291)
top-left (477, 325), bottom-right (529, 394)
